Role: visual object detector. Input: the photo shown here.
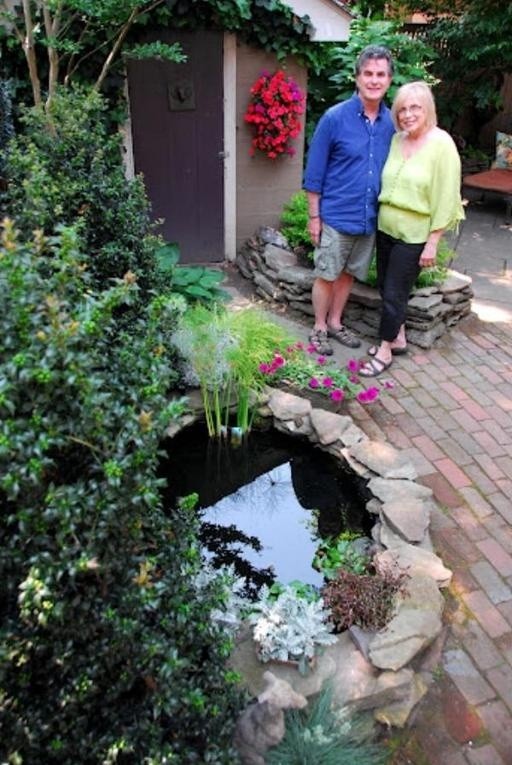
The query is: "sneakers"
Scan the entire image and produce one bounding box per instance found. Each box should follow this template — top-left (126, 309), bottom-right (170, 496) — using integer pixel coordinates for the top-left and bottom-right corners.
top-left (328, 325), bottom-right (360, 347)
top-left (309, 329), bottom-right (333, 355)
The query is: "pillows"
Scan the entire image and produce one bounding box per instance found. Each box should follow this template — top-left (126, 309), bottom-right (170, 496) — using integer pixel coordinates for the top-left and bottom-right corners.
top-left (489, 129), bottom-right (512, 174)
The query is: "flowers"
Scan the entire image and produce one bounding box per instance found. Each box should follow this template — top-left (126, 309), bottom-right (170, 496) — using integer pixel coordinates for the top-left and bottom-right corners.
top-left (246, 65), bottom-right (306, 164)
top-left (263, 342), bottom-right (396, 404)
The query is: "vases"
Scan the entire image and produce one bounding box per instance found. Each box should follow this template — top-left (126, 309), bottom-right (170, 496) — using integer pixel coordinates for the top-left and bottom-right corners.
top-left (277, 375), bottom-right (343, 416)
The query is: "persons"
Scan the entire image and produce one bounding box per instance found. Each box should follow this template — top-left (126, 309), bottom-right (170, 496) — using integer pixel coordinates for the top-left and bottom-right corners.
top-left (299, 43), bottom-right (397, 357)
top-left (359, 81), bottom-right (467, 378)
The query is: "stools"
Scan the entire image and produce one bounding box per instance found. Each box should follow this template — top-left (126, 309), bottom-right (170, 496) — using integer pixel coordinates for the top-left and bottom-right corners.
top-left (460, 160), bottom-right (512, 226)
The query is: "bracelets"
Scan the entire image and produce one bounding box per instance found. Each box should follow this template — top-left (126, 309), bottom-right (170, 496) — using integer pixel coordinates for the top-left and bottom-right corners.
top-left (307, 211), bottom-right (321, 221)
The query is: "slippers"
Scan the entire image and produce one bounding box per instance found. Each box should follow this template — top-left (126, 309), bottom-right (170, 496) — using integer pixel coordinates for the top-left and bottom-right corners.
top-left (359, 344), bottom-right (407, 377)
